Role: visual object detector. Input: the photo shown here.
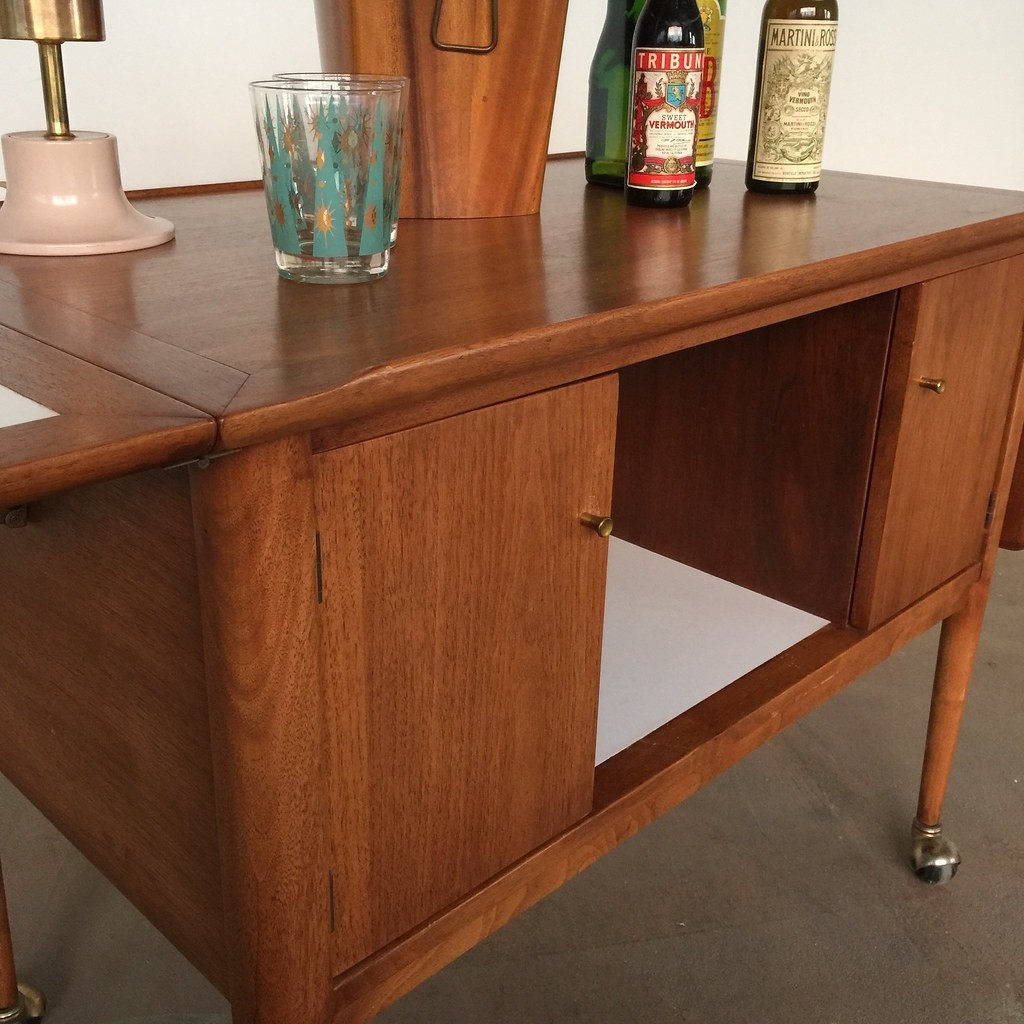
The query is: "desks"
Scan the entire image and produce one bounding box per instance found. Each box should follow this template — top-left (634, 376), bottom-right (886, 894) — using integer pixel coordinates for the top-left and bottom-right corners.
top-left (0, 150), bottom-right (1024, 1024)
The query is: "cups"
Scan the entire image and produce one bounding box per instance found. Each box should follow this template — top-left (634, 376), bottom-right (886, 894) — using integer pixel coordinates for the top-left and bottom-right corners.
top-left (248, 80), bottom-right (402, 284)
top-left (275, 70), bottom-right (409, 251)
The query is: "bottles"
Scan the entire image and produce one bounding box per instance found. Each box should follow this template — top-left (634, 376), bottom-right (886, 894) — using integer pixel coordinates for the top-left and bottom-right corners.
top-left (745, 0), bottom-right (838, 197)
top-left (623, 0), bottom-right (705, 209)
top-left (693, 0), bottom-right (726, 190)
top-left (584, 0), bottom-right (634, 187)
